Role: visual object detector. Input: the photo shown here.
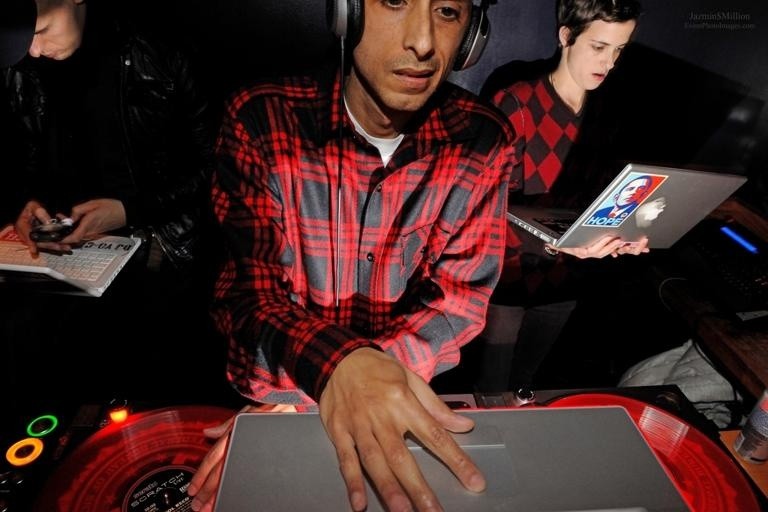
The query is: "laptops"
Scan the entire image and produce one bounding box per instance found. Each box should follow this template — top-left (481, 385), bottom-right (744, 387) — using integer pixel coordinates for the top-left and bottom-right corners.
top-left (212, 404), bottom-right (693, 511)
top-left (506, 161), bottom-right (748, 253)
top-left (0, 223), bottom-right (143, 299)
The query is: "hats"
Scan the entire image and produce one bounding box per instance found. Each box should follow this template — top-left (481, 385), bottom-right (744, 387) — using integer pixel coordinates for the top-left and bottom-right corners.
top-left (0, 1), bottom-right (37, 70)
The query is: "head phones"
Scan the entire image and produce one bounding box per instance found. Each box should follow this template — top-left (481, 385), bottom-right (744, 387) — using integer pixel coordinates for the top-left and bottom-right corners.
top-left (325, 0), bottom-right (493, 71)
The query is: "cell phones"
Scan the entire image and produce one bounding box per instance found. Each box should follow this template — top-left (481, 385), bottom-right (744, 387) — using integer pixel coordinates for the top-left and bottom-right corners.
top-left (29, 217), bottom-right (78, 241)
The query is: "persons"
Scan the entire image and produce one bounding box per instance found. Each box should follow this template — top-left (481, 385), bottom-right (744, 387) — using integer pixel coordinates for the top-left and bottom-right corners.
top-left (187, 0), bottom-right (517, 512)
top-left (0, 0), bottom-right (222, 417)
top-left (585, 175), bottom-right (652, 227)
top-left (481, 1), bottom-right (652, 397)
